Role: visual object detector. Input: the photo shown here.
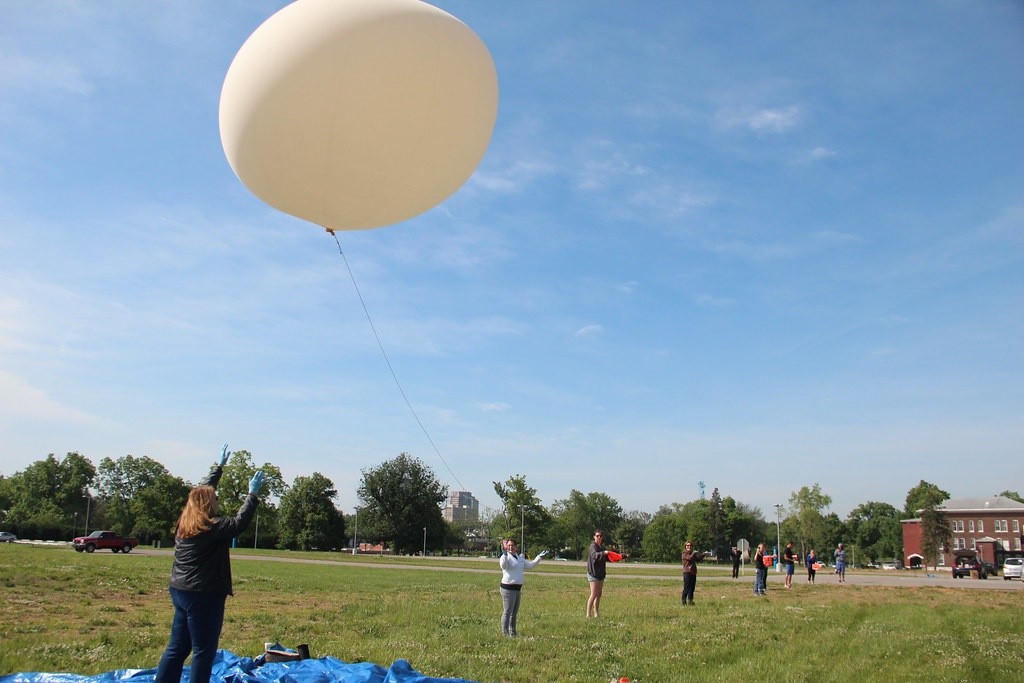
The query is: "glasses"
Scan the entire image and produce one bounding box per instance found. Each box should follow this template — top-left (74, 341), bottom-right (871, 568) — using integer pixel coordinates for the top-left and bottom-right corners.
top-left (686, 545), bottom-right (692, 547)
top-left (595, 534), bottom-right (602, 538)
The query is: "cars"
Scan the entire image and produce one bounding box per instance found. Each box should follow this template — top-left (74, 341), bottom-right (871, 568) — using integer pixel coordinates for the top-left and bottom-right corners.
top-left (951, 549), bottom-right (997, 579)
top-left (1002, 557), bottom-right (1024, 580)
top-left (0, 531), bottom-right (16, 543)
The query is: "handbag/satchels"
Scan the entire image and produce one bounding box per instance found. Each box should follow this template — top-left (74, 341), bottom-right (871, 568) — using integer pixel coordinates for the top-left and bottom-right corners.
top-left (812, 564), bottom-right (821, 570)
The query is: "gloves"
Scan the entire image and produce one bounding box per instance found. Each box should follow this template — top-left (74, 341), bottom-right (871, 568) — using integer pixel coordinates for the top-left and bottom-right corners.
top-left (218, 442), bottom-right (231, 465)
top-left (501, 544), bottom-right (508, 556)
top-left (538, 550), bottom-right (549, 559)
top-left (248, 470), bottom-right (267, 494)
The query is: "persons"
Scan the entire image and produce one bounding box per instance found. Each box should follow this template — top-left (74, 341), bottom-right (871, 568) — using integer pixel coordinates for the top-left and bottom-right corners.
top-left (156, 444), bottom-right (264, 682)
top-left (682, 540), bottom-right (711, 606)
top-left (784, 541), bottom-right (800, 588)
top-left (729, 547), bottom-right (743, 579)
top-left (585, 529), bottom-right (623, 621)
top-left (500, 534), bottom-right (549, 637)
top-left (807, 548), bottom-right (818, 584)
top-left (753, 543), bottom-right (778, 596)
top-left (833, 543), bottom-right (847, 583)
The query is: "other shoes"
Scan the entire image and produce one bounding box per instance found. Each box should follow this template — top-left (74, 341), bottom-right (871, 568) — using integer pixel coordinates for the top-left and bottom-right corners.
top-left (784, 584), bottom-right (793, 588)
top-left (808, 581), bottom-right (814, 585)
top-left (754, 594), bottom-right (766, 596)
top-left (839, 580), bottom-right (846, 583)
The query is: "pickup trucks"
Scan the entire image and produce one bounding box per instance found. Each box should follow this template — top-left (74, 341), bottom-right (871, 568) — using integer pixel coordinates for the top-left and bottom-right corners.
top-left (72, 530), bottom-right (140, 553)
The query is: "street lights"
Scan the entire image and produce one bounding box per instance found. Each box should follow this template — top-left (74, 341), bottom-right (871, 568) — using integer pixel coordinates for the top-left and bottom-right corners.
top-left (353, 505), bottom-right (360, 549)
top-left (516, 503), bottom-right (528, 554)
top-left (773, 504), bottom-right (783, 563)
